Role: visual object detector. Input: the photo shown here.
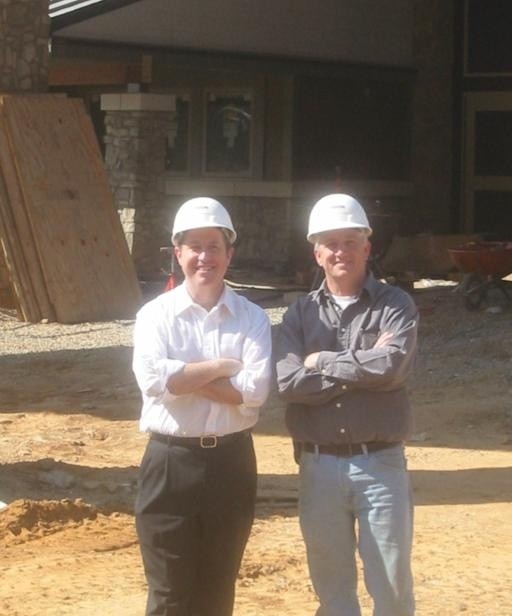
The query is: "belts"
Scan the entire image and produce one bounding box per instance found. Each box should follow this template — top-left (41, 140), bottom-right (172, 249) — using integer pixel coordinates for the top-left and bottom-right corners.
top-left (151, 431), bottom-right (249, 449)
top-left (301, 441), bottom-right (395, 456)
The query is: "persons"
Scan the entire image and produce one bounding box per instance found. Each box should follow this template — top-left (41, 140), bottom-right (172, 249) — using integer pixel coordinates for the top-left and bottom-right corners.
top-left (133, 197), bottom-right (272, 616)
top-left (272, 193), bottom-right (419, 616)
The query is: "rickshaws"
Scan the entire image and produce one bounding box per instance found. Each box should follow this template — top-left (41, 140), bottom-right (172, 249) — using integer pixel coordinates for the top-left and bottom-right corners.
top-left (447, 240), bottom-right (512, 311)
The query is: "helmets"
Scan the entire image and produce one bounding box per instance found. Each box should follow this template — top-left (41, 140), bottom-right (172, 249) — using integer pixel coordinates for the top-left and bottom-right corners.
top-left (306, 193), bottom-right (372, 245)
top-left (171, 197), bottom-right (237, 245)
